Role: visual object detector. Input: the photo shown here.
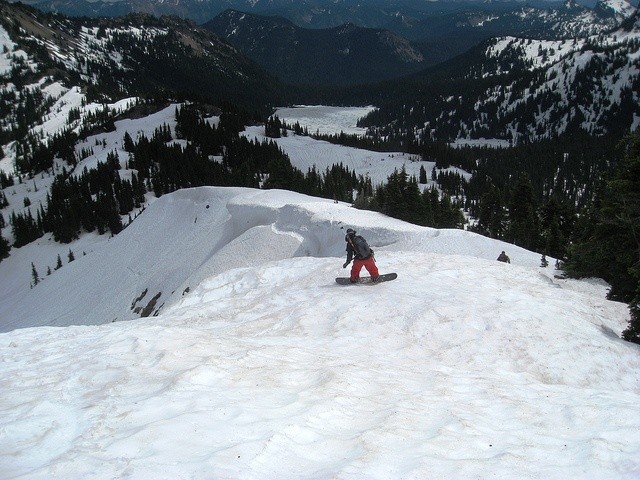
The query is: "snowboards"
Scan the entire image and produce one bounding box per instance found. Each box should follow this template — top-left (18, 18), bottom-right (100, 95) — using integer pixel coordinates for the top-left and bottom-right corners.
top-left (335, 272), bottom-right (397, 285)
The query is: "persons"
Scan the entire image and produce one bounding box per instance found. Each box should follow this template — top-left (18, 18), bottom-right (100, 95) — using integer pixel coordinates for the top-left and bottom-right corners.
top-left (541, 255), bottom-right (549, 266)
top-left (342, 228), bottom-right (382, 282)
top-left (496, 250), bottom-right (511, 264)
